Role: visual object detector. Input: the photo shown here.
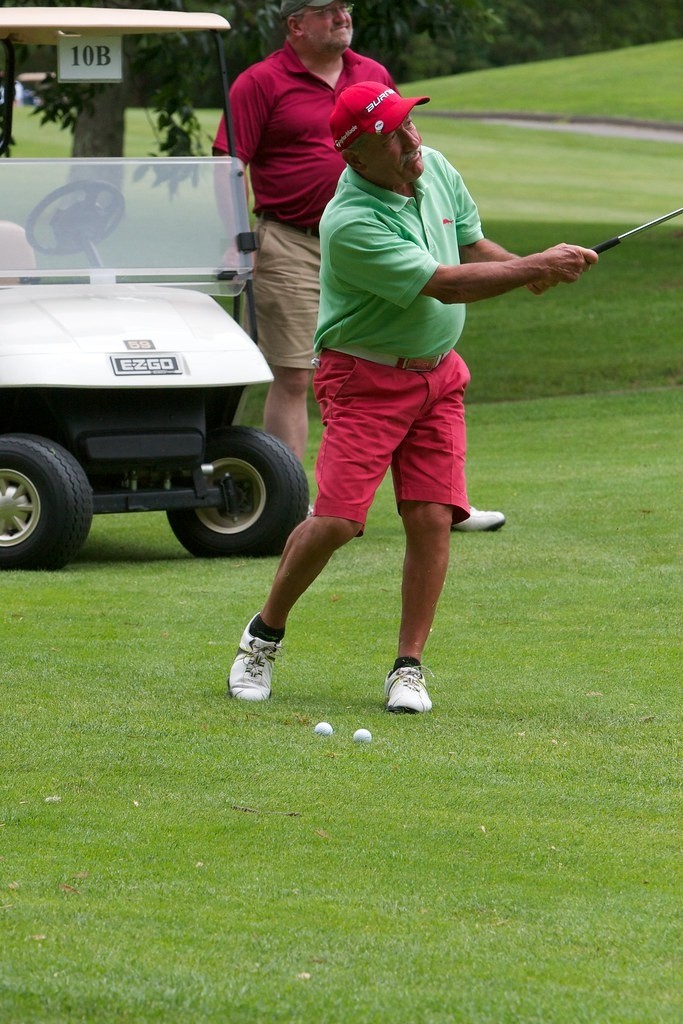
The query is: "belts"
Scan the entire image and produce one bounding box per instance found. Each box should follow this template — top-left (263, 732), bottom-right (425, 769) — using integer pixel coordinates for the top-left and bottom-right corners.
top-left (327, 345), bottom-right (451, 372)
top-left (254, 212), bottom-right (320, 237)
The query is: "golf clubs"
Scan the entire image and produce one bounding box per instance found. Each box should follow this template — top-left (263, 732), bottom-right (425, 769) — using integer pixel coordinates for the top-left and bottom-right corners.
top-left (590, 208), bottom-right (683, 256)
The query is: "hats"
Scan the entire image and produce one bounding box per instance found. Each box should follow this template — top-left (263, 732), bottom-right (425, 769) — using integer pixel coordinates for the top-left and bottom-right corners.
top-left (328, 81), bottom-right (431, 153)
top-left (280, 0), bottom-right (335, 17)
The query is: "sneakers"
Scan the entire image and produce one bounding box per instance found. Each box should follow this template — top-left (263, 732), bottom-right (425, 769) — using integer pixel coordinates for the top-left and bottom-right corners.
top-left (227, 612), bottom-right (284, 700)
top-left (451, 504), bottom-right (505, 532)
top-left (384, 665), bottom-right (435, 713)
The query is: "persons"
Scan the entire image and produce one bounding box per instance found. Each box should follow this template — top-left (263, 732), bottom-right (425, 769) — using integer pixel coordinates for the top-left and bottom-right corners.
top-left (211, 0), bottom-right (506, 531)
top-left (227, 84), bottom-right (598, 714)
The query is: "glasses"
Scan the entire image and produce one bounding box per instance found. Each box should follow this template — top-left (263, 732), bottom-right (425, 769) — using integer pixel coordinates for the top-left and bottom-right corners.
top-left (290, 3), bottom-right (355, 16)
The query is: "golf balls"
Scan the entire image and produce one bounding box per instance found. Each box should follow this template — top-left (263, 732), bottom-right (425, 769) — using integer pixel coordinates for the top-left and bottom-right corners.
top-left (353, 729), bottom-right (372, 743)
top-left (315, 721), bottom-right (334, 737)
